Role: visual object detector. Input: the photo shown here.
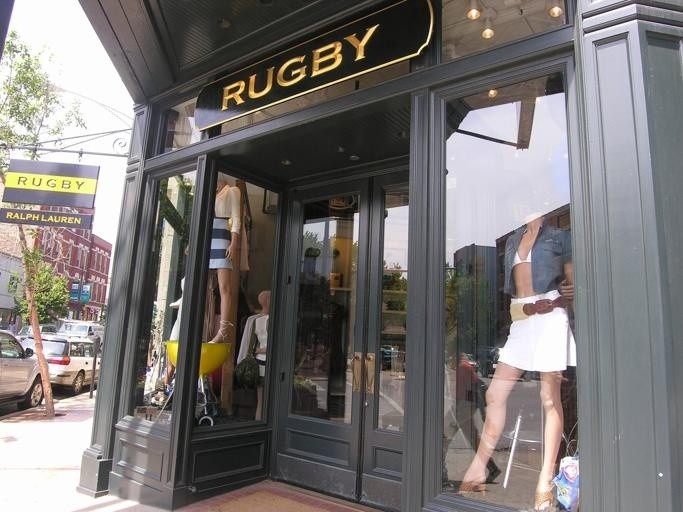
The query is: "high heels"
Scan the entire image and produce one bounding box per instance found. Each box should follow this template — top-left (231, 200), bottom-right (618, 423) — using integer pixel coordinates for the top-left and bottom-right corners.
top-left (450, 473), bottom-right (487, 498)
top-left (535, 483), bottom-right (556, 512)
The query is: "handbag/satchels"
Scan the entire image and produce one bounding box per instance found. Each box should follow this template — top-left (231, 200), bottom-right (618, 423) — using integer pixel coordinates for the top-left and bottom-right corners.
top-left (477, 379), bottom-right (488, 407)
top-left (235, 352), bottom-right (260, 391)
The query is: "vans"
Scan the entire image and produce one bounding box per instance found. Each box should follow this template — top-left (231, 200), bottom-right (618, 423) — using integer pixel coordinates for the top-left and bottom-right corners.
top-left (19, 333), bottom-right (103, 395)
top-left (58, 322), bottom-right (104, 348)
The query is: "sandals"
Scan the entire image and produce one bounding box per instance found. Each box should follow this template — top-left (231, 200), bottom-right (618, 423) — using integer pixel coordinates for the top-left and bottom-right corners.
top-left (208, 320), bottom-right (235, 345)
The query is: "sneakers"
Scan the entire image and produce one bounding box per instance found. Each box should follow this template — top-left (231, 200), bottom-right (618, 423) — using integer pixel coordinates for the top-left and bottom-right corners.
top-left (482, 469), bottom-right (501, 484)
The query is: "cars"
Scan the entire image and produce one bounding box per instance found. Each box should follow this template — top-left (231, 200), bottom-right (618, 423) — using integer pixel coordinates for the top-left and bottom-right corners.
top-left (0, 329), bottom-right (48, 410)
top-left (304, 346), bottom-right (578, 444)
top-left (14, 324), bottom-right (56, 343)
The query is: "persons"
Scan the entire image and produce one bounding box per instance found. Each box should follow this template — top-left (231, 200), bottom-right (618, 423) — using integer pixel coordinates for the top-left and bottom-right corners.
top-left (456, 212), bottom-right (575, 512)
top-left (180, 172), bottom-right (241, 343)
top-left (7, 321), bottom-right (16, 334)
top-left (441, 332), bottom-right (500, 490)
top-left (152, 276), bottom-right (186, 402)
top-left (236, 289), bottom-right (270, 422)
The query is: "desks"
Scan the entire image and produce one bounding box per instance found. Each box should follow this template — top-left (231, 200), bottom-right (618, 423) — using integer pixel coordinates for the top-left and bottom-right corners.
top-left (155, 342), bottom-right (232, 426)
top-left (486, 375), bottom-right (575, 491)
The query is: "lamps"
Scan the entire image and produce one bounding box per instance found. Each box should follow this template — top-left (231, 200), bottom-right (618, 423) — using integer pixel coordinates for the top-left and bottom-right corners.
top-left (545, 1), bottom-right (564, 18)
top-left (466, 0), bottom-right (480, 20)
top-left (481, 8), bottom-right (496, 40)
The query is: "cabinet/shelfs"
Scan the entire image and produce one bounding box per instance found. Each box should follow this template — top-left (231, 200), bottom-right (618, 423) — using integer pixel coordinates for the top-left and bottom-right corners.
top-left (330, 263), bottom-right (407, 345)
